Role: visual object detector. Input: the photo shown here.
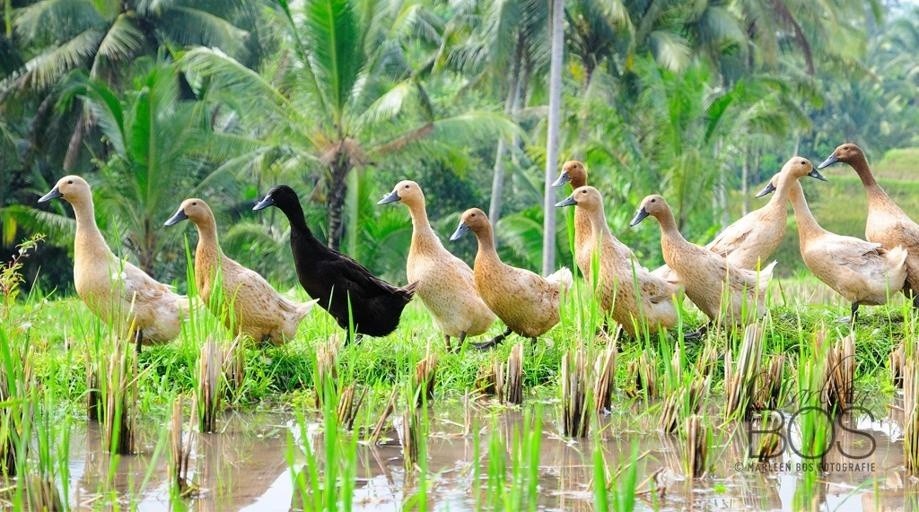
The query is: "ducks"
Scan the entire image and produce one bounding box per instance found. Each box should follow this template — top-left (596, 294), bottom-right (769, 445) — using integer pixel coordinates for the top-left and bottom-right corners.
top-left (818, 142), bottom-right (919, 313)
top-left (449, 207), bottom-right (573, 357)
top-left (554, 185), bottom-right (686, 352)
top-left (629, 194), bottom-right (778, 342)
top-left (252, 183), bottom-right (420, 348)
top-left (377, 179), bottom-right (497, 355)
top-left (551, 160), bottom-right (640, 334)
top-left (755, 172), bottom-right (909, 331)
top-left (37, 174), bottom-right (195, 354)
top-left (163, 197), bottom-right (321, 357)
top-left (700, 156), bottom-right (828, 274)
